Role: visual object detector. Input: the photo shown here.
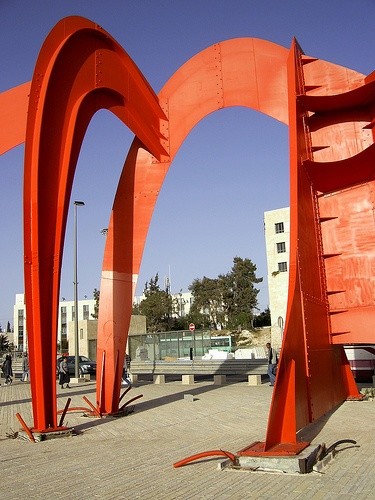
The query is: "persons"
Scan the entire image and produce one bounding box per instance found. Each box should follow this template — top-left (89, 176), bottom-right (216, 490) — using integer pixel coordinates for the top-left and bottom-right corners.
top-left (122, 359), bottom-right (131, 385)
top-left (21, 355), bottom-right (27, 382)
top-left (266, 342), bottom-right (277, 386)
top-left (1, 355), bottom-right (13, 384)
top-left (57, 355), bottom-right (71, 389)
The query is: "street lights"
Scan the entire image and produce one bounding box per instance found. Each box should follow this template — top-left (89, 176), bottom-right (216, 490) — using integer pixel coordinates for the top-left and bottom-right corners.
top-left (73, 200), bottom-right (86, 378)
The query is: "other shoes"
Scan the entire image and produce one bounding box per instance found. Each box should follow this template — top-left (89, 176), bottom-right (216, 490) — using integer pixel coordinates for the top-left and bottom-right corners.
top-left (66, 384), bottom-right (71, 388)
top-left (61, 385), bottom-right (66, 389)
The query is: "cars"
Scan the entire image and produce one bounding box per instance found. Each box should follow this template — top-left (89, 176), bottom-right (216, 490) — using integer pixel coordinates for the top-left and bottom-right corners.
top-left (57, 355), bottom-right (96, 376)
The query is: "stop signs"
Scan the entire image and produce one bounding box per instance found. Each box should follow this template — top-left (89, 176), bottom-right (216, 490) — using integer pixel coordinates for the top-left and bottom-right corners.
top-left (188, 322), bottom-right (195, 332)
